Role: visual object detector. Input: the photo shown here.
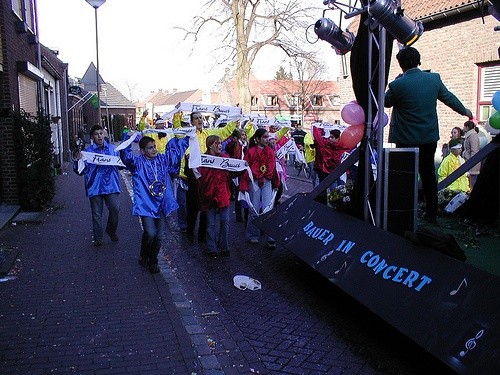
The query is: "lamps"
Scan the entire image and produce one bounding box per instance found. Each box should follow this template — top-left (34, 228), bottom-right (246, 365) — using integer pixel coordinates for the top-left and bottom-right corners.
top-left (315, 9), bottom-right (356, 55)
top-left (370, 0), bottom-right (425, 48)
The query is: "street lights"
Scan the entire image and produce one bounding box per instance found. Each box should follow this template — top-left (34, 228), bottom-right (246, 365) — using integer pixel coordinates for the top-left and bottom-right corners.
top-left (86, 0), bottom-right (106, 126)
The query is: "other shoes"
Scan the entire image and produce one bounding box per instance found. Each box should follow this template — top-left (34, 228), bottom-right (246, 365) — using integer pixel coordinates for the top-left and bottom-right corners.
top-left (149, 263), bottom-right (160, 273)
top-left (209, 251), bottom-right (218, 257)
top-left (222, 251), bottom-right (231, 256)
top-left (139, 256), bottom-right (149, 266)
top-left (267, 242), bottom-right (276, 248)
top-left (94, 240), bottom-right (102, 246)
top-left (250, 239), bottom-right (259, 243)
top-left (107, 231), bottom-right (119, 241)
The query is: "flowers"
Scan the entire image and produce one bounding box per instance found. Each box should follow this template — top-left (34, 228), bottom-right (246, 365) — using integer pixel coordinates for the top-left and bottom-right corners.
top-left (328, 182), bottom-right (352, 207)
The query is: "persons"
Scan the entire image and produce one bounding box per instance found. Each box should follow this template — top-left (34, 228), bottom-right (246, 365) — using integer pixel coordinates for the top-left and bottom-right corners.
top-left (72, 125), bottom-right (129, 246)
top-left (122, 102), bottom-right (378, 259)
top-left (438, 120), bottom-right (481, 197)
top-left (103, 126), bottom-right (110, 143)
top-left (120, 130), bottom-right (195, 273)
top-left (384, 47), bottom-right (473, 223)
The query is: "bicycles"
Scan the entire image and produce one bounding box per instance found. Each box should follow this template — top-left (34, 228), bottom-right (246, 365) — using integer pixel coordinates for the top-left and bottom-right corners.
top-left (293, 145), bottom-right (313, 179)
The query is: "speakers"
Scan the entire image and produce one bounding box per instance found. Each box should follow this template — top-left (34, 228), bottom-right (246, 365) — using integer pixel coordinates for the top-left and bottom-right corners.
top-left (466, 133), bottom-right (500, 227)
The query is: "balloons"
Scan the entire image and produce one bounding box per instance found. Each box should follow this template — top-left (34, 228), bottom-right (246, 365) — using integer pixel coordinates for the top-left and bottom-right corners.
top-left (341, 100), bottom-right (388, 146)
top-left (484, 91), bottom-right (500, 134)
